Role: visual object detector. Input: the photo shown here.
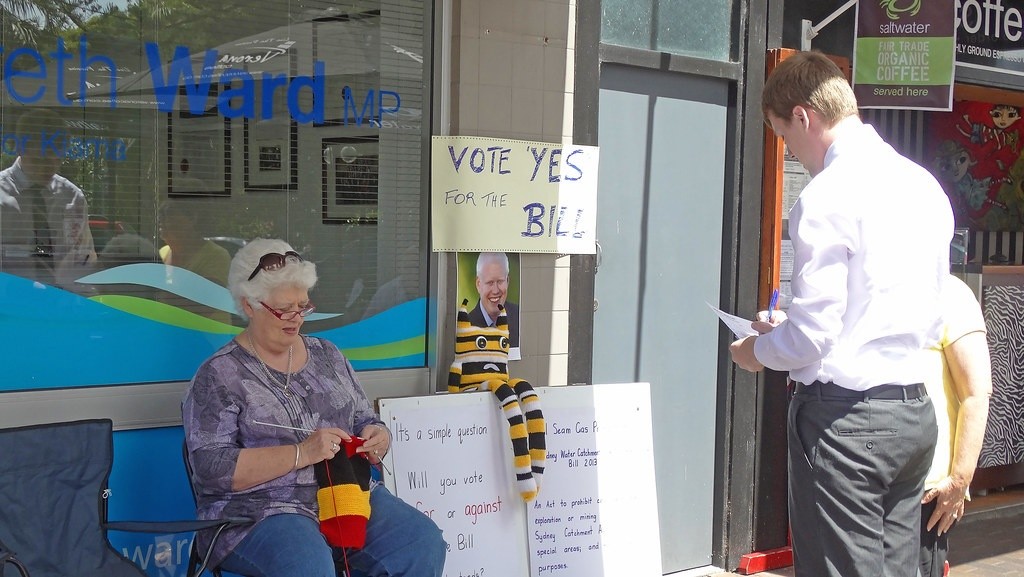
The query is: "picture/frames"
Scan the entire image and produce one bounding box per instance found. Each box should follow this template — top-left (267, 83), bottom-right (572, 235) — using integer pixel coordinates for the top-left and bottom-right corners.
top-left (311, 10), bottom-right (381, 128)
top-left (167, 53), bottom-right (232, 198)
top-left (242, 48), bottom-right (298, 192)
top-left (321, 135), bottom-right (380, 225)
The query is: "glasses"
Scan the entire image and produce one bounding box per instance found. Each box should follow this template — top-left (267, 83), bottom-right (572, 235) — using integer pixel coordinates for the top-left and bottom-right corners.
top-left (248, 251), bottom-right (304, 281)
top-left (258, 300), bottom-right (315, 321)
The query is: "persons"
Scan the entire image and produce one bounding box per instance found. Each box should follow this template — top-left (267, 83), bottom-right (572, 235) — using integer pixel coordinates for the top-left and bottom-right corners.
top-left (342, 230), bottom-right (421, 326)
top-left (182, 236), bottom-right (445, 577)
top-left (729, 52), bottom-right (955, 577)
top-left (468, 252), bottom-right (519, 349)
top-left (917, 273), bottom-right (992, 576)
top-left (0, 108), bottom-right (235, 324)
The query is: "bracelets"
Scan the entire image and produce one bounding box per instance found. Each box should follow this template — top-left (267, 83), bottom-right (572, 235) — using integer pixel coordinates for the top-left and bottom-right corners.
top-left (290, 443), bottom-right (300, 473)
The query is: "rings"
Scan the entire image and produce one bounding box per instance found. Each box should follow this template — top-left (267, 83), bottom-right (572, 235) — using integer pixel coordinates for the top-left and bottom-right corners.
top-left (952, 513), bottom-right (958, 518)
top-left (331, 443), bottom-right (337, 452)
top-left (373, 449), bottom-right (379, 455)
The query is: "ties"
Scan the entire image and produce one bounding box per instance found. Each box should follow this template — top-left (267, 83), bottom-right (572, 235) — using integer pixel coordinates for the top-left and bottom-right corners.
top-left (29, 184), bottom-right (56, 286)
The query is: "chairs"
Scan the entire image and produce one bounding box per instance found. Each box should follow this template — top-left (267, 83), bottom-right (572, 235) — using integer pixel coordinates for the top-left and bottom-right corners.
top-left (0, 418), bottom-right (359, 577)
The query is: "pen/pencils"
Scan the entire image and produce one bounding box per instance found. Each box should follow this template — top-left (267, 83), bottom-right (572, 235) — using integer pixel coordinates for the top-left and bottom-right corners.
top-left (767, 289), bottom-right (779, 319)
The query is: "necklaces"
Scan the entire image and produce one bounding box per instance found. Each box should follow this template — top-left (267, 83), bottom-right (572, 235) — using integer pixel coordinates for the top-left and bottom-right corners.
top-left (245, 327), bottom-right (293, 398)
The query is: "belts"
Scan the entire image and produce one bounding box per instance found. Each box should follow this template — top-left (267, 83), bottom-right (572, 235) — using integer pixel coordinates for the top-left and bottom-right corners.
top-left (794, 381), bottom-right (926, 399)
